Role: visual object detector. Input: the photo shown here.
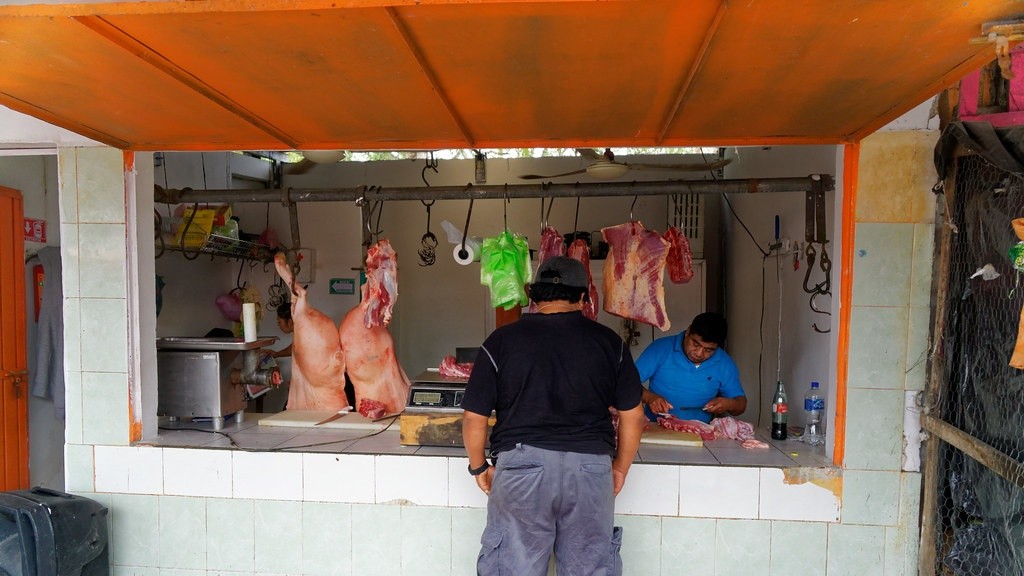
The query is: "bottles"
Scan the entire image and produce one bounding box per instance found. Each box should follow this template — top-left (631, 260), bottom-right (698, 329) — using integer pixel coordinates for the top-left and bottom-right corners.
top-left (771, 380), bottom-right (789, 441)
top-left (803, 382), bottom-right (825, 446)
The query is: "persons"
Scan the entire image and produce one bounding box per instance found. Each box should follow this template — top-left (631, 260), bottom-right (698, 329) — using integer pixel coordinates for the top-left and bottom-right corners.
top-left (634, 313), bottom-right (747, 424)
top-left (262, 306), bottom-right (294, 357)
top-left (461, 256), bottom-right (645, 575)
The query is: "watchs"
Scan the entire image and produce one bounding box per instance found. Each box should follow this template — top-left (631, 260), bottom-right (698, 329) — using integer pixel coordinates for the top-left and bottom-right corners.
top-left (467, 462), bottom-right (488, 476)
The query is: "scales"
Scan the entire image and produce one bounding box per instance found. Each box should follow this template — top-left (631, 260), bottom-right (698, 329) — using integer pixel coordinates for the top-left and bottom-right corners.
top-left (404, 367), bottom-right (497, 414)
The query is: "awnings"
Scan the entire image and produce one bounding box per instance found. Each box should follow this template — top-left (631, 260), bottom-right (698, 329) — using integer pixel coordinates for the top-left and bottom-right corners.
top-left (2, 0), bottom-right (1024, 157)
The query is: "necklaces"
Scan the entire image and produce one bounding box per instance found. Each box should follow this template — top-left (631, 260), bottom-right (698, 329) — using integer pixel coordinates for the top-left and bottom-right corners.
top-left (532, 303), bottom-right (576, 313)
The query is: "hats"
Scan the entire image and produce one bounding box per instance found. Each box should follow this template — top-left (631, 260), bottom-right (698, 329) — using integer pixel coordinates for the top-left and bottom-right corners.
top-left (535, 254), bottom-right (586, 287)
